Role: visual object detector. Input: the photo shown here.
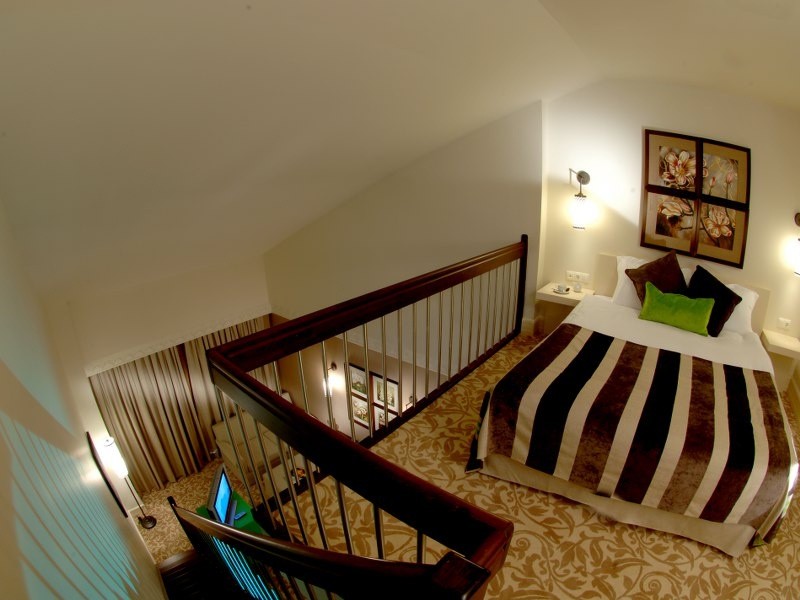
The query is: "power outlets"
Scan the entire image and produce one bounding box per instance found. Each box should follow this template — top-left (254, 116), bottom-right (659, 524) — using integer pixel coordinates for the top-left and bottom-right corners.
top-left (566, 270), bottom-right (593, 285)
top-left (777, 317), bottom-right (791, 329)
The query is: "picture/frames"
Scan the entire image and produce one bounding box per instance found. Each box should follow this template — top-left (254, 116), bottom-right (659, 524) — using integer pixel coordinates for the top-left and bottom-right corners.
top-left (350, 392), bottom-right (370, 430)
top-left (693, 195), bottom-right (749, 269)
top-left (698, 136), bottom-right (753, 208)
top-left (344, 361), bottom-right (370, 401)
top-left (640, 190), bottom-right (700, 257)
top-left (369, 369), bottom-right (401, 415)
top-left (644, 129), bottom-right (700, 198)
top-left (372, 402), bottom-right (398, 431)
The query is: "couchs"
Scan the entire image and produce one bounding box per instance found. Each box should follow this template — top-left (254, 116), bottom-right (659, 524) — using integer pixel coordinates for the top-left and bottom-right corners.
top-left (212, 392), bottom-right (301, 487)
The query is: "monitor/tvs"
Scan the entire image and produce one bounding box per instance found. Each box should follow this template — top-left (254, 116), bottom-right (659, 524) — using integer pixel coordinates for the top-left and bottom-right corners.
top-left (205, 463), bottom-right (238, 527)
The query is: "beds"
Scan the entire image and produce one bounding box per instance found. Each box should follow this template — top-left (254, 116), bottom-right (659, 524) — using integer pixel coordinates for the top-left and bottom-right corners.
top-left (475, 292), bottom-right (800, 558)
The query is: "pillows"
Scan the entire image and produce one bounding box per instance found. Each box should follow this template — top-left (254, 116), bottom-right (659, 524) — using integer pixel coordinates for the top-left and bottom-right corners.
top-left (719, 283), bottom-right (760, 333)
top-left (684, 264), bottom-right (743, 337)
top-left (638, 280), bottom-right (715, 337)
top-left (625, 248), bottom-right (688, 309)
top-left (609, 256), bottom-right (652, 310)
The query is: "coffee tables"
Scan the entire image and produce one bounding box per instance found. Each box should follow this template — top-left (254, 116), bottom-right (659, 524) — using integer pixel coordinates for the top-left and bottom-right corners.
top-left (262, 453), bottom-right (319, 498)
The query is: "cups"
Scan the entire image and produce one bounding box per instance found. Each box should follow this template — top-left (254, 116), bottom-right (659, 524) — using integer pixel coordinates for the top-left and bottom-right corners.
top-left (558, 285), bottom-right (566, 292)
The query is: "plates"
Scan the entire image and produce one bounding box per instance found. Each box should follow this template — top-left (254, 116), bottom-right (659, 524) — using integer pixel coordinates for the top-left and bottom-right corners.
top-left (554, 288), bottom-right (569, 293)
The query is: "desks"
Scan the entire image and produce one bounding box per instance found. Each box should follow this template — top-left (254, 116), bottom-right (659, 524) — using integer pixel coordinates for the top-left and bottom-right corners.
top-left (195, 491), bottom-right (272, 537)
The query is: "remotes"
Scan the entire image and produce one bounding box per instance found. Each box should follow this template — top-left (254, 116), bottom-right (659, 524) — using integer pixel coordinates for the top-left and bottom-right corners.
top-left (233, 511), bottom-right (246, 520)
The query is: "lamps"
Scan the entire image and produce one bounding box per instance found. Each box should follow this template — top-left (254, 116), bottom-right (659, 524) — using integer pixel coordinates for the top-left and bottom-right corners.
top-left (569, 168), bottom-right (590, 231)
top-left (101, 436), bottom-right (157, 529)
top-left (323, 362), bottom-right (338, 399)
top-left (792, 212), bottom-right (800, 277)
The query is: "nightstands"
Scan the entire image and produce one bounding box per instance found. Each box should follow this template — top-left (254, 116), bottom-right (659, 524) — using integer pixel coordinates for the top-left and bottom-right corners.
top-left (535, 281), bottom-right (595, 336)
top-left (759, 329), bottom-right (800, 398)
